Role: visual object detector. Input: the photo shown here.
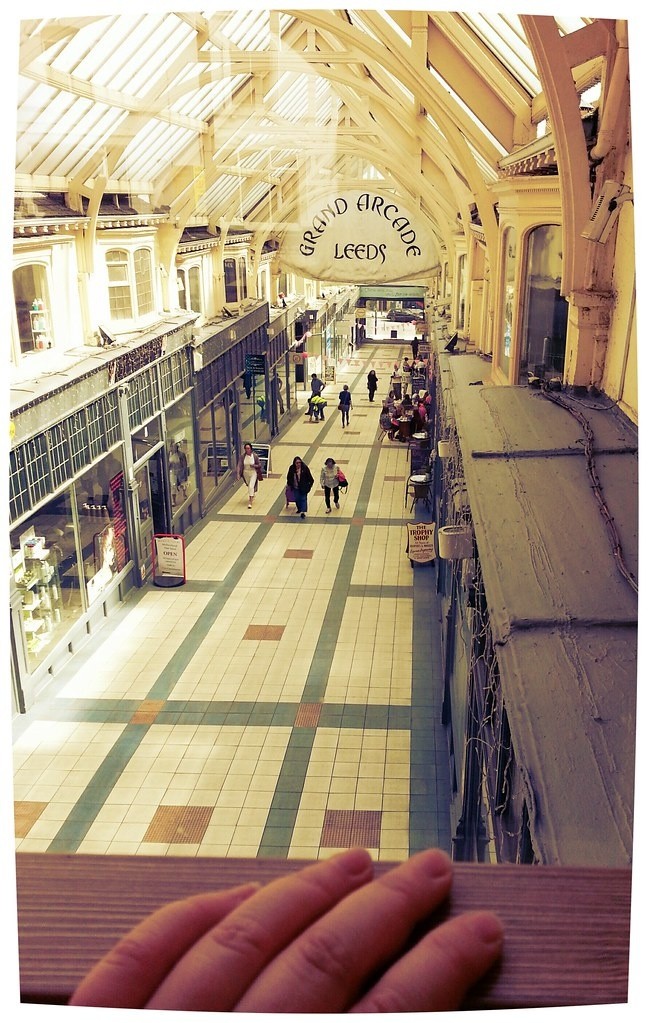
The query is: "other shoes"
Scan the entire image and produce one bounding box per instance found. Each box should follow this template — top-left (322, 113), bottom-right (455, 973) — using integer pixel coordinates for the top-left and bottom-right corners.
top-left (334, 500), bottom-right (339, 508)
top-left (301, 513), bottom-right (306, 518)
top-left (248, 504), bottom-right (252, 508)
top-left (297, 510), bottom-right (301, 513)
top-left (326, 508), bottom-right (332, 513)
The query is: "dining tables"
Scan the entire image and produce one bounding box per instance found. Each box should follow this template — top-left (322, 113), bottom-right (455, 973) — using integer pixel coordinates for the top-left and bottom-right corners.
top-left (396, 414), bottom-right (415, 442)
top-left (393, 398), bottom-right (418, 411)
top-left (410, 474), bottom-right (433, 504)
top-left (406, 432), bottom-right (431, 459)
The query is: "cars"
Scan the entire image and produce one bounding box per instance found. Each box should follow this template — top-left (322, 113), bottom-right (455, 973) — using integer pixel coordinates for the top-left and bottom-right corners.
top-left (386, 308), bottom-right (420, 323)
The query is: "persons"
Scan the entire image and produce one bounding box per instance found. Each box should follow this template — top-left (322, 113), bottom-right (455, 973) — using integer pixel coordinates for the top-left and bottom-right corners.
top-left (306, 396), bottom-right (329, 425)
top-left (85, 526), bottom-right (119, 607)
top-left (60, 845), bottom-right (515, 1014)
top-left (416, 398), bottom-right (426, 425)
top-left (414, 354), bottom-right (425, 361)
top-left (412, 393), bottom-right (421, 409)
top-left (365, 370), bottom-right (380, 402)
top-left (414, 358), bottom-right (427, 378)
top-left (380, 406), bottom-right (397, 439)
top-left (239, 370), bottom-right (259, 402)
top-left (319, 457), bottom-right (345, 514)
top-left (270, 371), bottom-right (286, 415)
top-left (287, 455), bottom-right (314, 520)
top-left (164, 442), bottom-right (192, 507)
top-left (423, 390), bottom-right (432, 409)
top-left (234, 442), bottom-right (264, 509)
top-left (402, 356), bottom-right (412, 374)
top-left (338, 384), bottom-right (355, 430)
top-left (410, 361), bottom-right (418, 381)
top-left (400, 394), bottom-right (412, 411)
top-left (408, 409), bottom-right (420, 440)
top-left (383, 390), bottom-right (399, 417)
top-left (388, 363), bottom-right (404, 401)
top-left (390, 402), bottom-right (405, 428)
top-left (304, 373), bottom-right (328, 415)
top-left (409, 336), bottom-right (421, 360)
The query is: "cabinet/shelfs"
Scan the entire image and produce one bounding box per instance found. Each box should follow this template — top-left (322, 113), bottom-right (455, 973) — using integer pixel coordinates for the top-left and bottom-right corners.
top-left (28, 309), bottom-right (53, 353)
top-left (9, 503), bottom-right (114, 659)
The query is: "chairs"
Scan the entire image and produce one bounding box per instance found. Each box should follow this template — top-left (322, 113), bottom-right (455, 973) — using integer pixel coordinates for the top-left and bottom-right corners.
top-left (403, 405), bottom-right (414, 418)
top-left (377, 419), bottom-right (394, 442)
top-left (404, 470), bottom-right (429, 508)
top-left (410, 484), bottom-right (431, 514)
top-left (405, 421), bottom-right (424, 444)
top-left (406, 427), bottom-right (429, 462)
top-left (411, 439), bottom-right (431, 460)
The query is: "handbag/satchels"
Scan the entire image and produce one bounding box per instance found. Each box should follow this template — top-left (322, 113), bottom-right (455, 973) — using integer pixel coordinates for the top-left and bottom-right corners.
top-left (298, 482), bottom-right (311, 493)
top-left (338, 479), bottom-right (348, 494)
top-left (337, 470), bottom-right (345, 482)
top-left (338, 406), bottom-right (342, 410)
